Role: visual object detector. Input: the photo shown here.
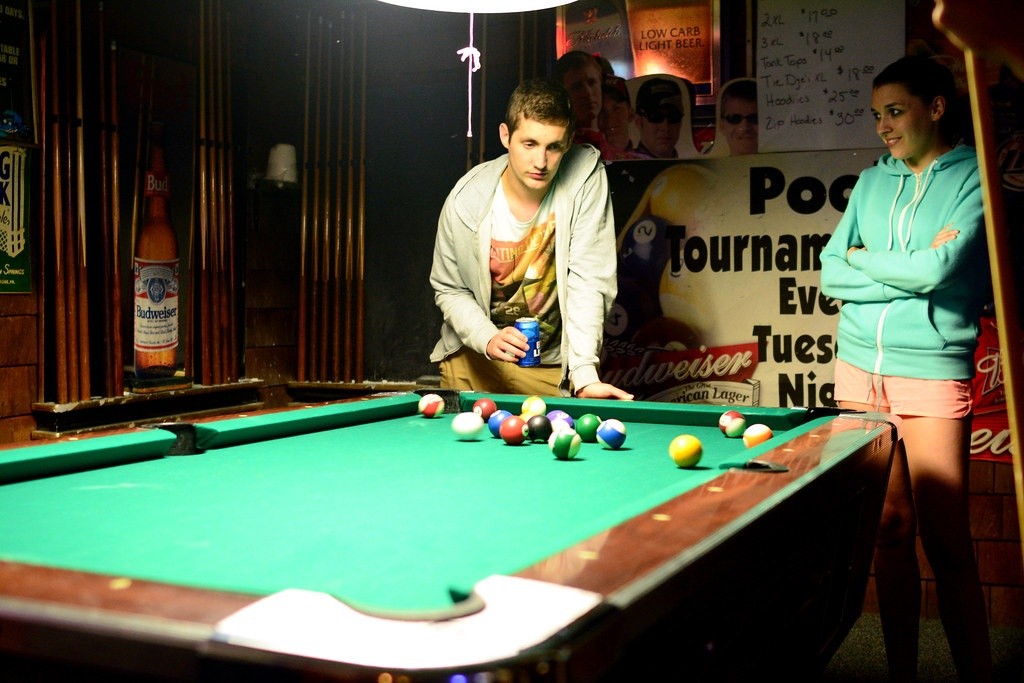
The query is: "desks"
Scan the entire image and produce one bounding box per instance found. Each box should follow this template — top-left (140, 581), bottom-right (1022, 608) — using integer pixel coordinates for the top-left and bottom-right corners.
top-left (0, 375), bottom-right (904, 682)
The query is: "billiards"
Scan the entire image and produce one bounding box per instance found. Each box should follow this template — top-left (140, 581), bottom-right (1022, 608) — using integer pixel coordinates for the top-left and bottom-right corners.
top-left (415, 392), bottom-right (776, 470)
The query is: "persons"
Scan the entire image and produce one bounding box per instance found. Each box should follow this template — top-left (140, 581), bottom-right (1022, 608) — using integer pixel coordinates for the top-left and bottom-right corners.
top-left (818, 57), bottom-right (992, 683)
top-left (559, 52), bottom-right (759, 163)
top-left (430, 75), bottom-right (636, 401)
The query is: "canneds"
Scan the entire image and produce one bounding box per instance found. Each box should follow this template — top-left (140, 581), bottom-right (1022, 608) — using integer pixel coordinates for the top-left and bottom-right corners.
top-left (514, 317), bottom-right (542, 369)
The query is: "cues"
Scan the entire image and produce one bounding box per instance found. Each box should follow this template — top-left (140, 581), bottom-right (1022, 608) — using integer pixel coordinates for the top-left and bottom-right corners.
top-left (464, 11), bottom-right (543, 177)
top-left (967, 49), bottom-right (1024, 569)
top-left (30, 0), bottom-right (372, 415)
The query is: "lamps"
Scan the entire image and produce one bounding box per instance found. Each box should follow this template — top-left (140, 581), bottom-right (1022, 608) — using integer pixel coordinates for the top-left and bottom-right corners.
top-left (247, 142), bottom-right (299, 190)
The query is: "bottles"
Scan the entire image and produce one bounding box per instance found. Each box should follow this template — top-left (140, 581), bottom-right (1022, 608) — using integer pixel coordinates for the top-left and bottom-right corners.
top-left (133, 121), bottom-right (181, 379)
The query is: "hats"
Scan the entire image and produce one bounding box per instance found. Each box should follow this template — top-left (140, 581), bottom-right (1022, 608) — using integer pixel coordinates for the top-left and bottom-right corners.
top-left (601, 77), bottom-right (630, 101)
top-left (635, 79), bottom-right (685, 115)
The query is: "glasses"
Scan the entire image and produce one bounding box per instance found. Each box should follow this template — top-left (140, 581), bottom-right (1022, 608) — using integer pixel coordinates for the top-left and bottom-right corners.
top-left (723, 113), bottom-right (758, 125)
top-left (642, 110), bottom-right (682, 124)
